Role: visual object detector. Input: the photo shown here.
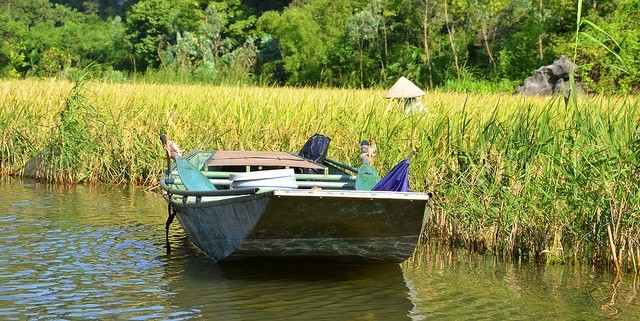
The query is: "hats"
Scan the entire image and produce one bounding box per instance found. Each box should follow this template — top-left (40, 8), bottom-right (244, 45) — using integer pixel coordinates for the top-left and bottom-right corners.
top-left (382, 76), bottom-right (425, 99)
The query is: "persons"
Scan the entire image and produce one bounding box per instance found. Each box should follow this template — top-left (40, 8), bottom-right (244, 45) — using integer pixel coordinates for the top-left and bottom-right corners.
top-left (397, 98), bottom-right (429, 115)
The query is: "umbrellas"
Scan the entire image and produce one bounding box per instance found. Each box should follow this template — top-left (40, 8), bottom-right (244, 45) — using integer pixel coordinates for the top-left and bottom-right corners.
top-left (370, 149), bottom-right (416, 191)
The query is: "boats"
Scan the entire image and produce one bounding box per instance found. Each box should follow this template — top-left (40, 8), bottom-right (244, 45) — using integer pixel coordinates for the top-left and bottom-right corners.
top-left (159, 150), bottom-right (428, 262)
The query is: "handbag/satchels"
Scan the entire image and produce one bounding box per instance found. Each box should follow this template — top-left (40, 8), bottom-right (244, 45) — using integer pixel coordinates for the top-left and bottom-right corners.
top-left (298, 133), bottom-right (331, 165)
